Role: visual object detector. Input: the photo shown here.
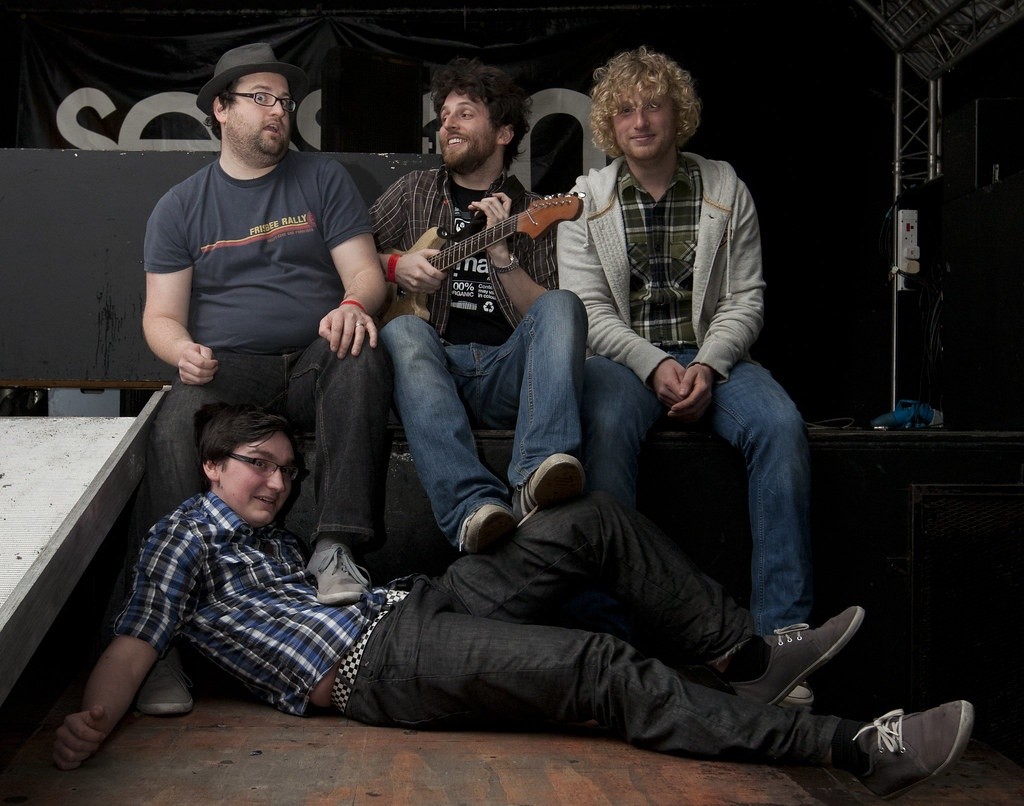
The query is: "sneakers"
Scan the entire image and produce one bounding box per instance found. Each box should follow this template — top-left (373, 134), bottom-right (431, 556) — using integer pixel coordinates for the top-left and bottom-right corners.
top-left (852, 700), bottom-right (974, 801)
top-left (512, 452), bottom-right (586, 528)
top-left (731, 606), bottom-right (864, 706)
top-left (459, 505), bottom-right (516, 555)
top-left (777, 678), bottom-right (815, 711)
top-left (304, 541), bottom-right (371, 605)
top-left (135, 647), bottom-right (194, 716)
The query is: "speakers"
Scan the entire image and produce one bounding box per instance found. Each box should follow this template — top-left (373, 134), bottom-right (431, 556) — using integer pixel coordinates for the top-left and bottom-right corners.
top-left (900, 483), bottom-right (1024, 759)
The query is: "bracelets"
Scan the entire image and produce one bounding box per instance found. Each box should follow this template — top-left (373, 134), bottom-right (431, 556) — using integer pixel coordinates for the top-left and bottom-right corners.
top-left (386, 254), bottom-right (399, 283)
top-left (341, 301), bottom-right (367, 314)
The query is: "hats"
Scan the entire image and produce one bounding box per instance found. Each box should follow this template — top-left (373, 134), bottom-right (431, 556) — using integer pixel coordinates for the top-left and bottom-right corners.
top-left (195, 42), bottom-right (310, 116)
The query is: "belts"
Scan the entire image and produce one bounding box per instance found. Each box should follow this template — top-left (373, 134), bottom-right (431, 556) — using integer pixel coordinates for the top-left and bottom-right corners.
top-left (330, 591), bottom-right (409, 714)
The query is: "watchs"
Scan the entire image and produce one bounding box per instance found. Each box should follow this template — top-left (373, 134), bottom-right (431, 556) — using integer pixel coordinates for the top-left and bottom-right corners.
top-left (491, 254), bottom-right (519, 273)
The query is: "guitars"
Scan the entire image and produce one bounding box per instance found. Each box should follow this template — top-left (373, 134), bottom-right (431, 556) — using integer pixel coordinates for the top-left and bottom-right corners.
top-left (380, 190), bottom-right (585, 326)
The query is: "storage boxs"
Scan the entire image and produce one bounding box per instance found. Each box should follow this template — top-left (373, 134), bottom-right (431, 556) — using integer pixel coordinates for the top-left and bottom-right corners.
top-left (942, 96), bottom-right (1024, 203)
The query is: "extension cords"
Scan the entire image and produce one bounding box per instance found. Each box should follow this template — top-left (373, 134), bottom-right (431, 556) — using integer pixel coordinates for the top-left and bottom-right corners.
top-left (898, 209), bottom-right (920, 273)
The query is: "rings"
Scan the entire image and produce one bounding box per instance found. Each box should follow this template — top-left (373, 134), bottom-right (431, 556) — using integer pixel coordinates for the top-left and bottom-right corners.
top-left (356, 324), bottom-right (364, 327)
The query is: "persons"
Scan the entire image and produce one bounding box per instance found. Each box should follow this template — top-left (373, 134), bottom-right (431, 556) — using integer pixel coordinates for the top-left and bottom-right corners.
top-left (48, 405), bottom-right (973, 801)
top-left (366, 59), bottom-right (588, 554)
top-left (555, 47), bottom-right (815, 704)
top-left (131, 41), bottom-right (389, 713)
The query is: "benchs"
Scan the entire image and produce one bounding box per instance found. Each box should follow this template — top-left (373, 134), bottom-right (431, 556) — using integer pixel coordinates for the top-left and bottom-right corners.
top-left (282, 426), bottom-right (1024, 770)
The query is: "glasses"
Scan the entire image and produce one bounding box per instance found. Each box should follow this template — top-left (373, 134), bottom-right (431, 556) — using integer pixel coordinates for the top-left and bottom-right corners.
top-left (227, 91), bottom-right (295, 112)
top-left (226, 451), bottom-right (298, 480)
